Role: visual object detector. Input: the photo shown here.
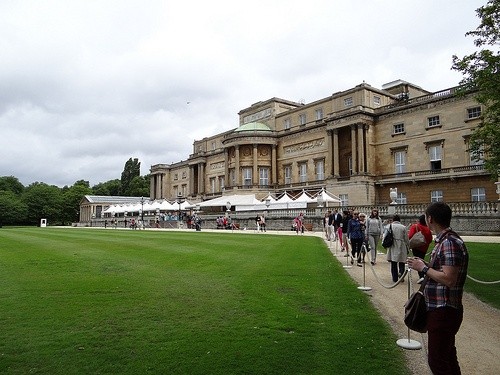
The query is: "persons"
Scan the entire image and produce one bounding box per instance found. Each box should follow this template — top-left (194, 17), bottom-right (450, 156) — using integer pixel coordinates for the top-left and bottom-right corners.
top-left (156, 218), bottom-right (159, 228)
top-left (292, 213), bottom-right (304, 233)
top-left (255, 213), bottom-right (267, 233)
top-left (186, 215), bottom-right (203, 229)
top-left (110, 218), bottom-right (135, 229)
top-left (216, 216), bottom-right (239, 230)
top-left (323, 209), bottom-right (393, 267)
top-left (408, 214), bottom-right (432, 284)
top-left (406, 201), bottom-right (469, 375)
top-left (382, 216), bottom-right (409, 284)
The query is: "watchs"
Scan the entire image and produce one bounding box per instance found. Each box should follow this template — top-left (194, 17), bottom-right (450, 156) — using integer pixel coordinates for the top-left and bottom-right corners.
top-left (422, 267), bottom-right (430, 274)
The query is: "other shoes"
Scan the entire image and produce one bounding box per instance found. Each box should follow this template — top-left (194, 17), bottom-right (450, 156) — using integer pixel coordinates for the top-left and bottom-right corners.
top-left (344, 253), bottom-right (349, 257)
top-left (371, 261), bottom-right (376, 265)
top-left (361, 260), bottom-right (365, 263)
top-left (351, 256), bottom-right (354, 263)
top-left (398, 273), bottom-right (404, 282)
top-left (341, 246), bottom-right (344, 252)
top-left (357, 263), bottom-right (362, 267)
top-left (417, 277), bottom-right (424, 283)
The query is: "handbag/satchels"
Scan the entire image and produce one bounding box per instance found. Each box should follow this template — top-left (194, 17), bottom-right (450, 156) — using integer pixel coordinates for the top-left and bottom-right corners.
top-left (382, 224), bottom-right (393, 248)
top-left (407, 224), bottom-right (425, 249)
top-left (403, 292), bottom-right (428, 333)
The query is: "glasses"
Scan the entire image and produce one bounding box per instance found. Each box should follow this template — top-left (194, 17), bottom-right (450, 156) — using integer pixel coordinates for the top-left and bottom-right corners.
top-left (360, 216), bottom-right (365, 217)
top-left (354, 214), bottom-right (358, 215)
